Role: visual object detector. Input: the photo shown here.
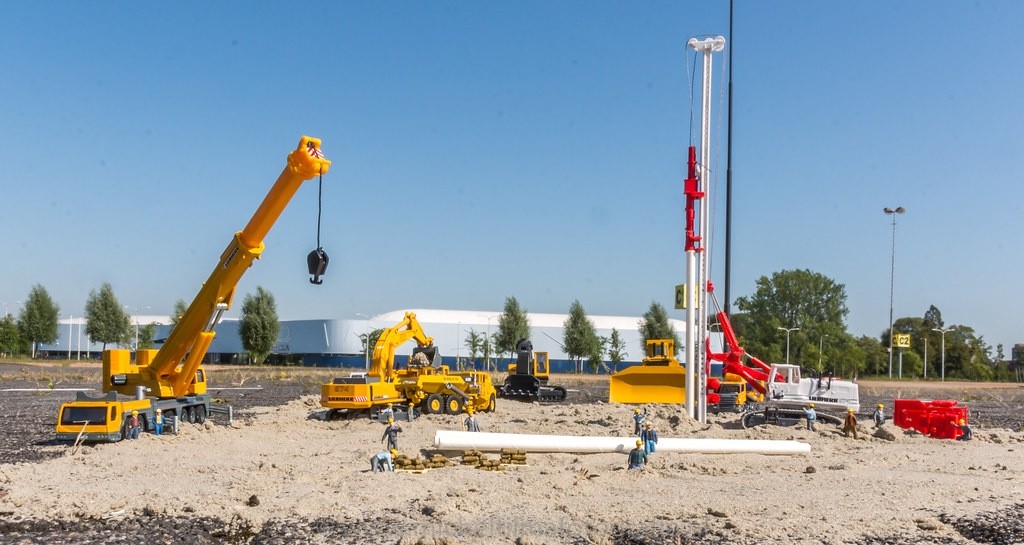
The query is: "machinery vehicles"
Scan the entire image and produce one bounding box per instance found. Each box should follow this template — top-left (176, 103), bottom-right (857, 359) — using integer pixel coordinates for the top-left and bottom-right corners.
top-left (53, 135), bottom-right (332, 450)
top-left (494, 337), bottom-right (568, 403)
top-left (607, 338), bottom-right (699, 406)
top-left (317, 310), bottom-right (444, 423)
top-left (680, 30), bottom-right (786, 425)
top-left (711, 360), bottom-right (862, 433)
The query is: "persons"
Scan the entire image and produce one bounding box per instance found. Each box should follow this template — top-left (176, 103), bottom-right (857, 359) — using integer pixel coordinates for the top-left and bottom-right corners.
top-left (407, 402), bottom-right (414, 422)
top-left (381, 416), bottom-right (402, 449)
top-left (634, 408), bottom-right (649, 436)
top-left (641, 423), bottom-right (658, 454)
top-left (371, 448), bottom-right (399, 473)
top-left (874, 403), bottom-right (885, 425)
top-left (380, 403), bottom-right (394, 419)
top-left (951, 419), bottom-right (973, 440)
top-left (127, 410), bottom-right (139, 439)
top-left (152, 408), bottom-right (166, 435)
top-left (628, 439), bottom-right (648, 469)
top-left (464, 411), bottom-right (481, 432)
top-left (845, 407), bottom-right (858, 439)
top-left (803, 402), bottom-right (817, 431)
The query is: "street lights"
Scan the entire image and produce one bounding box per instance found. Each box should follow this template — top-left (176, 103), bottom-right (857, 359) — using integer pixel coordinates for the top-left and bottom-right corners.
top-left (818, 334), bottom-right (829, 371)
top-left (124, 305), bottom-right (151, 363)
top-left (777, 327), bottom-right (800, 364)
top-left (883, 205), bottom-right (905, 381)
top-left (931, 328), bottom-right (955, 382)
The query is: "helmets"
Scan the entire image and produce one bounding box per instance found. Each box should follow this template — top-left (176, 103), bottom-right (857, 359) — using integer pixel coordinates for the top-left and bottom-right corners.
top-left (959, 419), bottom-right (964, 424)
top-left (157, 408), bottom-right (161, 412)
top-left (388, 418), bottom-right (393, 423)
top-left (636, 439), bottom-right (642, 445)
top-left (848, 408), bottom-right (853, 413)
top-left (878, 404), bottom-right (884, 408)
top-left (387, 403), bottom-right (393, 406)
top-left (635, 409), bottom-right (640, 414)
top-left (390, 449), bottom-right (398, 458)
top-left (132, 410), bottom-right (139, 415)
top-left (645, 421), bottom-right (652, 426)
top-left (809, 403), bottom-right (814, 408)
top-left (409, 402), bottom-right (415, 406)
top-left (468, 411), bottom-right (473, 415)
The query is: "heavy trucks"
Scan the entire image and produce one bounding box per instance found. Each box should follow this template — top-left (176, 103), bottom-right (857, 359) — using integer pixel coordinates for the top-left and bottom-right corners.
top-left (392, 367), bottom-right (497, 416)
